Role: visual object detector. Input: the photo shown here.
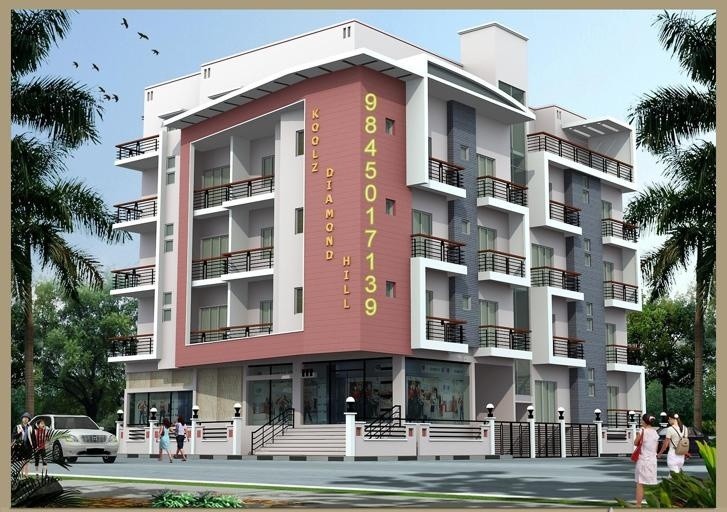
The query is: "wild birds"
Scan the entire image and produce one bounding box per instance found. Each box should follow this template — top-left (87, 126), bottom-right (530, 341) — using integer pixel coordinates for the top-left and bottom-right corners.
top-left (98, 87), bottom-right (105, 93)
top-left (73, 62), bottom-right (78, 68)
top-left (104, 95), bottom-right (110, 101)
top-left (112, 94), bottom-right (118, 102)
top-left (92, 64), bottom-right (99, 72)
top-left (152, 50), bottom-right (159, 56)
top-left (121, 18), bottom-right (128, 28)
top-left (138, 32), bottom-right (149, 40)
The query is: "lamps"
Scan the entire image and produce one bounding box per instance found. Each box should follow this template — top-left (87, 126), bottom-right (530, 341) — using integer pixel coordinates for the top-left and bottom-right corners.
top-left (191, 404), bottom-right (201, 418)
top-left (527, 404), bottom-right (536, 420)
top-left (116, 408), bottom-right (123, 421)
top-left (149, 407), bottom-right (159, 420)
top-left (595, 408), bottom-right (603, 420)
top-left (556, 406), bottom-right (565, 420)
top-left (345, 396), bottom-right (355, 413)
top-left (486, 401), bottom-right (494, 417)
top-left (628, 409), bottom-right (637, 421)
top-left (233, 402), bottom-right (243, 418)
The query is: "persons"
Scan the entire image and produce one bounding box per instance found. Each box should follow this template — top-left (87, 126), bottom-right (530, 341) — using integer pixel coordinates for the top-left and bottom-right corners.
top-left (158, 418), bottom-right (174, 463)
top-left (351, 382), bottom-right (380, 417)
top-left (172, 416), bottom-right (189, 459)
top-left (406, 381), bottom-right (441, 424)
top-left (656, 412), bottom-right (689, 479)
top-left (136, 398), bottom-right (166, 424)
top-left (262, 395), bottom-right (291, 422)
top-left (634, 413), bottom-right (660, 508)
top-left (12, 412), bottom-right (52, 481)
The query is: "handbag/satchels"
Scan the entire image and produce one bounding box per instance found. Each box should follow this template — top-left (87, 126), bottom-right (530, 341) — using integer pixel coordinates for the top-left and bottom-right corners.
top-left (631, 429), bottom-right (644, 462)
top-left (159, 426), bottom-right (165, 436)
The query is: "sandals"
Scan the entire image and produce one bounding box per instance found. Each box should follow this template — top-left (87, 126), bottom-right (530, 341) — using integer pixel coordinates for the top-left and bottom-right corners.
top-left (170, 454), bottom-right (187, 463)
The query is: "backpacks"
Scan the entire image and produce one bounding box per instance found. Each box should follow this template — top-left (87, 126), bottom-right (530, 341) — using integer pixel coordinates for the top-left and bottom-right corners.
top-left (670, 424), bottom-right (690, 455)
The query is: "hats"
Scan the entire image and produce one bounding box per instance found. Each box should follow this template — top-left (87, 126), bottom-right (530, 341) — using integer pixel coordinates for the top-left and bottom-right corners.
top-left (21, 412), bottom-right (32, 422)
